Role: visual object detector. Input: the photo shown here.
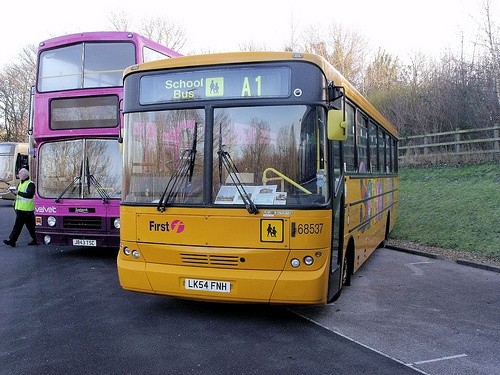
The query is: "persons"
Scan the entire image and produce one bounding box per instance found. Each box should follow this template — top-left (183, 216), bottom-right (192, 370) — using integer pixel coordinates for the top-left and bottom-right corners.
top-left (2, 168), bottom-right (41, 247)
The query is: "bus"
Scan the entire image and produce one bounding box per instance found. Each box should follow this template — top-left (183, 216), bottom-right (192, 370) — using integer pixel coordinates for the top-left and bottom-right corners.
top-left (28, 32), bottom-right (187, 245)
top-left (117, 51), bottom-right (399, 304)
top-left (0, 142), bottom-right (30, 200)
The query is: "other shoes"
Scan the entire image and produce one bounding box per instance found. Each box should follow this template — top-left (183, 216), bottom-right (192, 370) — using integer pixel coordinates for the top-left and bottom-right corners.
top-left (28, 241), bottom-right (39, 245)
top-left (3, 240), bottom-right (16, 247)
